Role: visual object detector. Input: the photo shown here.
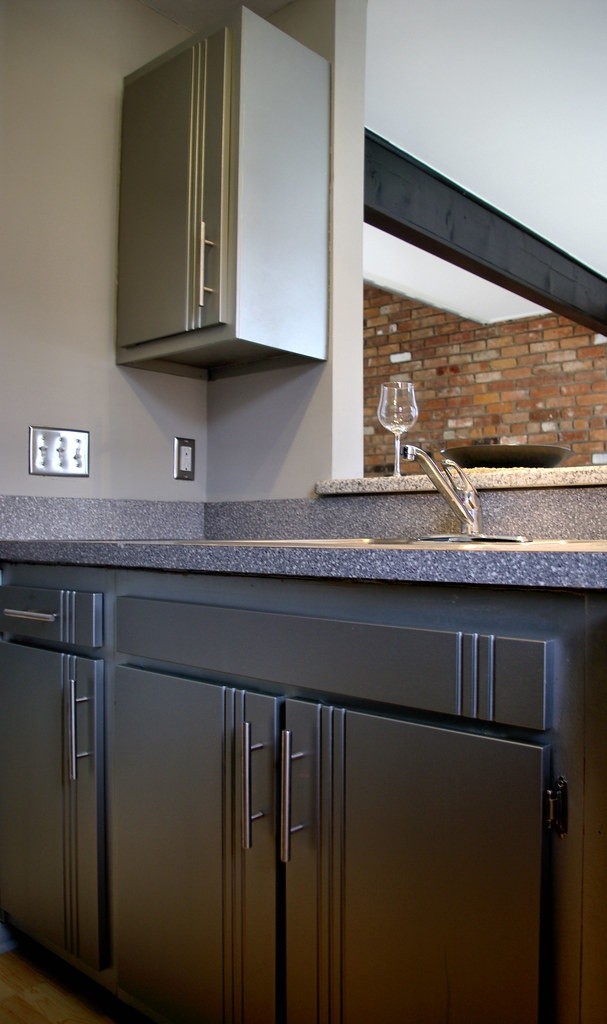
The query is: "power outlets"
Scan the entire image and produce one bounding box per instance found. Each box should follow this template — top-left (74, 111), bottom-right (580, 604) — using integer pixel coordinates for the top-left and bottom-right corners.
top-left (174, 437), bottom-right (196, 481)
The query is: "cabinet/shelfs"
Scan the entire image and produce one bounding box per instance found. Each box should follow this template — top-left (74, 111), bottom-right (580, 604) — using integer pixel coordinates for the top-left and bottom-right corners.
top-left (0, 560), bottom-right (115, 997)
top-left (115, 4), bottom-right (330, 382)
top-left (114, 566), bottom-right (607, 1024)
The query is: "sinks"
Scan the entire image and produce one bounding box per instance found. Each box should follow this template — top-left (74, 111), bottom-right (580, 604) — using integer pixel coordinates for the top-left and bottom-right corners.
top-left (177, 538), bottom-right (410, 549)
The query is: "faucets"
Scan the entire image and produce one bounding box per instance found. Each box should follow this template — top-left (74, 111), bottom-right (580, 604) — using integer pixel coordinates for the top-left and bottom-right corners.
top-left (402, 446), bottom-right (482, 535)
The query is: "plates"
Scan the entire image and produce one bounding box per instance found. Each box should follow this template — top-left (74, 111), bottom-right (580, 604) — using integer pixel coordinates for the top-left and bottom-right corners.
top-left (440, 444), bottom-right (571, 469)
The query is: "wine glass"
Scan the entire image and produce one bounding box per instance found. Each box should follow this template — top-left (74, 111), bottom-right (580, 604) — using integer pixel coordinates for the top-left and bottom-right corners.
top-left (377, 381), bottom-right (418, 476)
